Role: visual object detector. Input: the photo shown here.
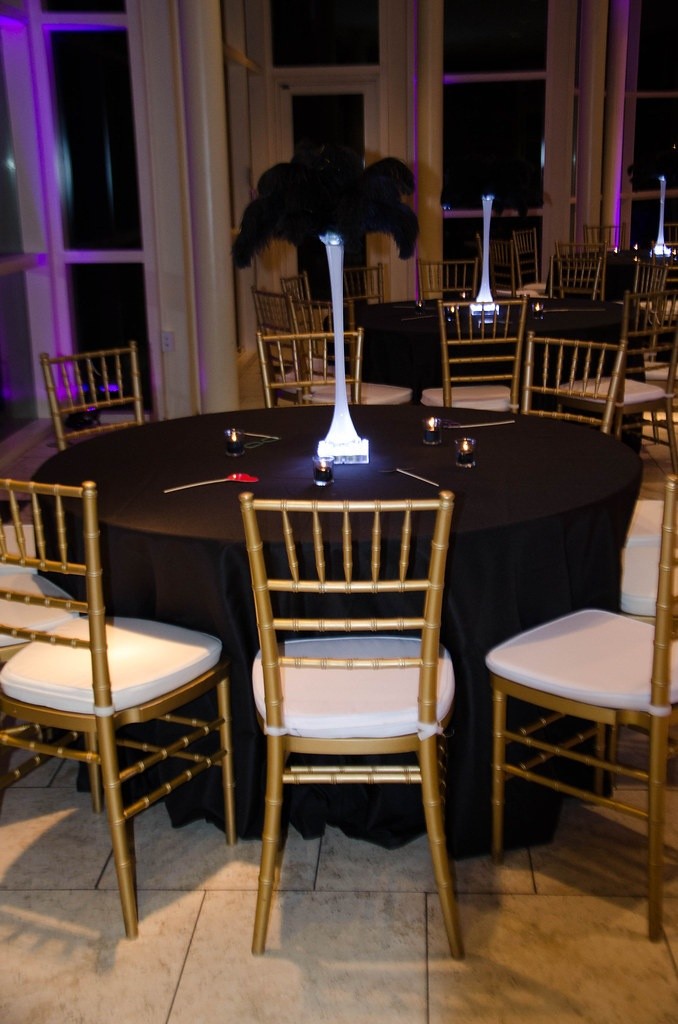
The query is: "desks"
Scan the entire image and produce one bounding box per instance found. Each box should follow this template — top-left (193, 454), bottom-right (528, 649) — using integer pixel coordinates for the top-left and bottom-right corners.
top-left (544, 246), bottom-right (678, 362)
top-left (30, 404), bottom-right (644, 860)
top-left (321, 296), bottom-right (653, 454)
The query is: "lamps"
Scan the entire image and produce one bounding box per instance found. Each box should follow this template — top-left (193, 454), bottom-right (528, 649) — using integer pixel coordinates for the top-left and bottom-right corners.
top-left (650, 176), bottom-right (671, 258)
top-left (316, 229), bottom-right (369, 464)
top-left (471, 193), bottom-right (500, 317)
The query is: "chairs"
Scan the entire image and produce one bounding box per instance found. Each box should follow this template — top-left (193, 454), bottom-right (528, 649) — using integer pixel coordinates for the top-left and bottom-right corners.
top-left (0, 228), bottom-right (678, 959)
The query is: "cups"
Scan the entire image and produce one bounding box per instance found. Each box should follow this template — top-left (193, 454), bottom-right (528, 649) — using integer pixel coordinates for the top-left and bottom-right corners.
top-left (415, 300), bottom-right (425, 315)
top-left (224, 429), bottom-right (245, 456)
top-left (533, 304), bottom-right (543, 320)
top-left (313, 456), bottom-right (335, 486)
top-left (456, 438), bottom-right (475, 468)
top-left (422, 417), bottom-right (442, 445)
